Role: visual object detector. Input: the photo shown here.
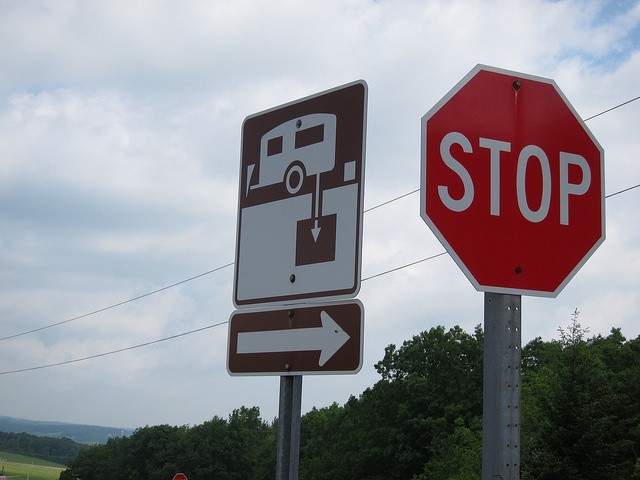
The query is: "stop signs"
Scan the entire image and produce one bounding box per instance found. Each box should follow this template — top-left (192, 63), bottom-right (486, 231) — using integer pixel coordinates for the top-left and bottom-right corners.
top-left (420, 64), bottom-right (606, 299)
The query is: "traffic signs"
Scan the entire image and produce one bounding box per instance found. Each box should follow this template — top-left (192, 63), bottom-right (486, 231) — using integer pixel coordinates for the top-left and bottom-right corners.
top-left (233, 79), bottom-right (368, 309)
